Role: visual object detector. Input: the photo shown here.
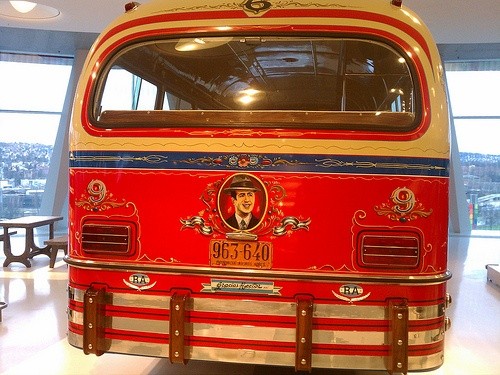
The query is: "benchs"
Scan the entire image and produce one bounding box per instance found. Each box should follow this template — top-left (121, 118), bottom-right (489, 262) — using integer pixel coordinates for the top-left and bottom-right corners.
top-left (44, 236), bottom-right (68, 268)
top-left (0, 230), bottom-right (17, 240)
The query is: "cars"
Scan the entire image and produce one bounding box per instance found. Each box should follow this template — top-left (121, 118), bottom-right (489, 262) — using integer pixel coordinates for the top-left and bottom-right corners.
top-left (467, 194), bottom-right (500, 220)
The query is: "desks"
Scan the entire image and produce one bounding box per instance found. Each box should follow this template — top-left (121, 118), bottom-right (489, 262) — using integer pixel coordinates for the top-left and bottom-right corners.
top-left (0, 216), bottom-right (64, 268)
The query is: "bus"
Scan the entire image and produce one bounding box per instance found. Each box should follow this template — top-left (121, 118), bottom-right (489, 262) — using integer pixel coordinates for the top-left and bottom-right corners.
top-left (64, 0), bottom-right (454, 375)
